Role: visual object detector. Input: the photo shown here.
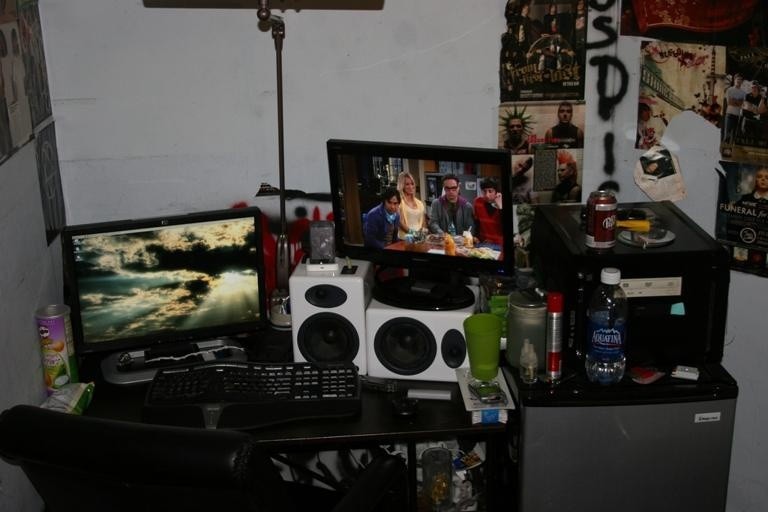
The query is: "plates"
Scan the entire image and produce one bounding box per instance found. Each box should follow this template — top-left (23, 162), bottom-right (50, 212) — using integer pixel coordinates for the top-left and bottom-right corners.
top-left (618, 227), bottom-right (676, 248)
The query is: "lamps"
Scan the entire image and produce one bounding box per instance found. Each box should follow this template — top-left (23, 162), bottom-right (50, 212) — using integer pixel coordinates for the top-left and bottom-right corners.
top-left (140, 2), bottom-right (383, 330)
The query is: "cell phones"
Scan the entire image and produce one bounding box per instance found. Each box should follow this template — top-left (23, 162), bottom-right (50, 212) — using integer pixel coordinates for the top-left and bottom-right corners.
top-left (470, 380), bottom-right (506, 403)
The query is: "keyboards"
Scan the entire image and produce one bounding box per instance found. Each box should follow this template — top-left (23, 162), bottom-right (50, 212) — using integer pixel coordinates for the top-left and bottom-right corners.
top-left (143, 362), bottom-right (362, 434)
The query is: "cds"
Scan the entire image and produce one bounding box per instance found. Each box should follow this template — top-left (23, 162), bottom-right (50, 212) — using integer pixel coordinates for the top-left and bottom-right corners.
top-left (619, 227), bottom-right (676, 249)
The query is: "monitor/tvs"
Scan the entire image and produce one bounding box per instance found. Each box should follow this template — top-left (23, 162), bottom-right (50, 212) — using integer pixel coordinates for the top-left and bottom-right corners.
top-left (326, 138), bottom-right (516, 312)
top-left (59, 207), bottom-right (268, 387)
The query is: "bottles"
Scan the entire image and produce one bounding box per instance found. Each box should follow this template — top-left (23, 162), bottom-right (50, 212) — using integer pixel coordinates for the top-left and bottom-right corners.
top-left (585, 268), bottom-right (625, 385)
top-left (507, 291), bottom-right (547, 368)
top-left (546, 292), bottom-right (563, 380)
top-left (36, 303), bottom-right (79, 397)
top-left (480, 272), bottom-right (515, 350)
top-left (586, 190), bottom-right (617, 249)
top-left (447, 221), bottom-right (456, 236)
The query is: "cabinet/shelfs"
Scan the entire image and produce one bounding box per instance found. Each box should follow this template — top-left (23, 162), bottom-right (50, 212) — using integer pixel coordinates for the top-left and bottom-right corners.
top-left (531, 201), bottom-right (733, 375)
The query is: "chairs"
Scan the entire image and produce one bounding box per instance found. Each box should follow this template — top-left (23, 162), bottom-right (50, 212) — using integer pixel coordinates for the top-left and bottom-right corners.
top-left (0, 402), bottom-right (409, 512)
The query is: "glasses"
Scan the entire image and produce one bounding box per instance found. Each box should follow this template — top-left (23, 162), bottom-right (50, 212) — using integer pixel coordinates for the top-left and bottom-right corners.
top-left (444, 185), bottom-right (459, 190)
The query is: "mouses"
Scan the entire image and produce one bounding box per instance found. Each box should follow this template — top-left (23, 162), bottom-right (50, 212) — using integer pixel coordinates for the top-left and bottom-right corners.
top-left (390, 396), bottom-right (420, 419)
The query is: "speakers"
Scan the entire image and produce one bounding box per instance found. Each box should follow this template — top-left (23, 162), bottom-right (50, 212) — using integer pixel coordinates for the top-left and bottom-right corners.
top-left (365, 286), bottom-right (481, 383)
top-left (288, 258), bottom-right (371, 376)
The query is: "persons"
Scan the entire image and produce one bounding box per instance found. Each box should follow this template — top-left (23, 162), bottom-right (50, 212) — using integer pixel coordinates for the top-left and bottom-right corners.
top-left (741, 81), bottom-right (768, 147)
top-left (639, 127), bottom-right (658, 149)
top-left (511, 155), bottom-right (533, 205)
top-left (428, 175), bottom-right (475, 238)
top-left (395, 171), bottom-right (426, 242)
top-left (637, 101), bottom-right (666, 141)
top-left (363, 190), bottom-right (400, 249)
top-left (552, 152), bottom-right (581, 204)
top-left (544, 102), bottom-right (583, 147)
top-left (499, 106), bottom-right (533, 154)
top-left (723, 74), bottom-right (746, 145)
top-left (473, 177), bottom-right (504, 249)
top-left (734, 169), bottom-right (768, 211)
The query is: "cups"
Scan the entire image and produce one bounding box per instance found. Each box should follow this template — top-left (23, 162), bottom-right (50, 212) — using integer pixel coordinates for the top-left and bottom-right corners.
top-left (421, 447), bottom-right (454, 512)
top-left (464, 312), bottom-right (507, 383)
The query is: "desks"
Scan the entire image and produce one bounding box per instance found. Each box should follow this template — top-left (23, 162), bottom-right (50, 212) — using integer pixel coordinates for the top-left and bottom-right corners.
top-left (38, 327), bottom-right (520, 512)
